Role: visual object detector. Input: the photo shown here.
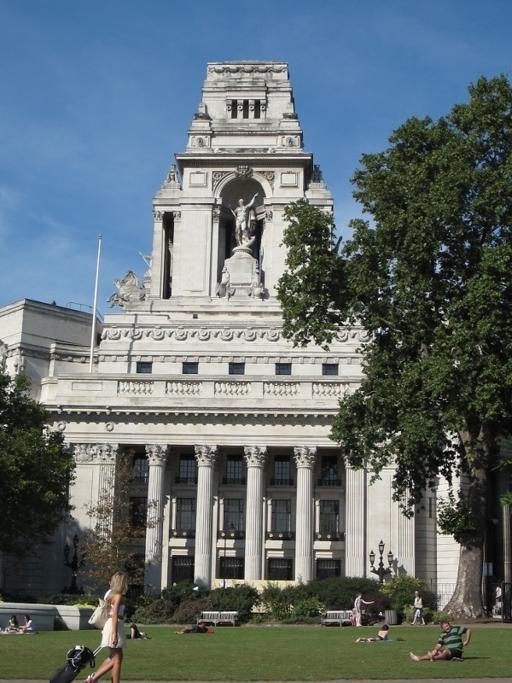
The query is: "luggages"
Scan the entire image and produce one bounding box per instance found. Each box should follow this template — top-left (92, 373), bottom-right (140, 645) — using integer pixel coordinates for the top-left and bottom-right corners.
top-left (49, 646), bottom-right (103, 683)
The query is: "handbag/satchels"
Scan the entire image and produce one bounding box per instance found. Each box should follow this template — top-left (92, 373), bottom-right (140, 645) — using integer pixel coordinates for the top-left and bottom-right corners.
top-left (65, 644), bottom-right (95, 668)
top-left (88, 598), bottom-right (111, 629)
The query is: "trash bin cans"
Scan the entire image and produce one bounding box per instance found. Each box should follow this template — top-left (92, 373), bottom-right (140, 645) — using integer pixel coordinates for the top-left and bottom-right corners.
top-left (384, 610), bottom-right (398, 625)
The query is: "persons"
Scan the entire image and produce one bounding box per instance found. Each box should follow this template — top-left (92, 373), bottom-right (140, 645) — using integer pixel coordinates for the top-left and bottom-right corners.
top-left (128, 622), bottom-right (149, 640)
top-left (6, 614), bottom-right (19, 632)
top-left (173, 622), bottom-right (210, 635)
top-left (228, 189), bottom-right (261, 250)
top-left (17, 612), bottom-right (33, 634)
top-left (410, 590), bottom-right (425, 625)
top-left (408, 619), bottom-right (471, 662)
top-left (350, 606), bottom-right (355, 626)
top-left (354, 624), bottom-right (390, 642)
top-left (353, 591), bottom-right (376, 627)
top-left (84, 571), bottom-right (130, 683)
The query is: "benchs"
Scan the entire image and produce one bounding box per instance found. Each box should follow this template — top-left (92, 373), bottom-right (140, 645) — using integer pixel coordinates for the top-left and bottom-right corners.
top-left (321, 610), bottom-right (352, 627)
top-left (197, 611), bottom-right (239, 628)
